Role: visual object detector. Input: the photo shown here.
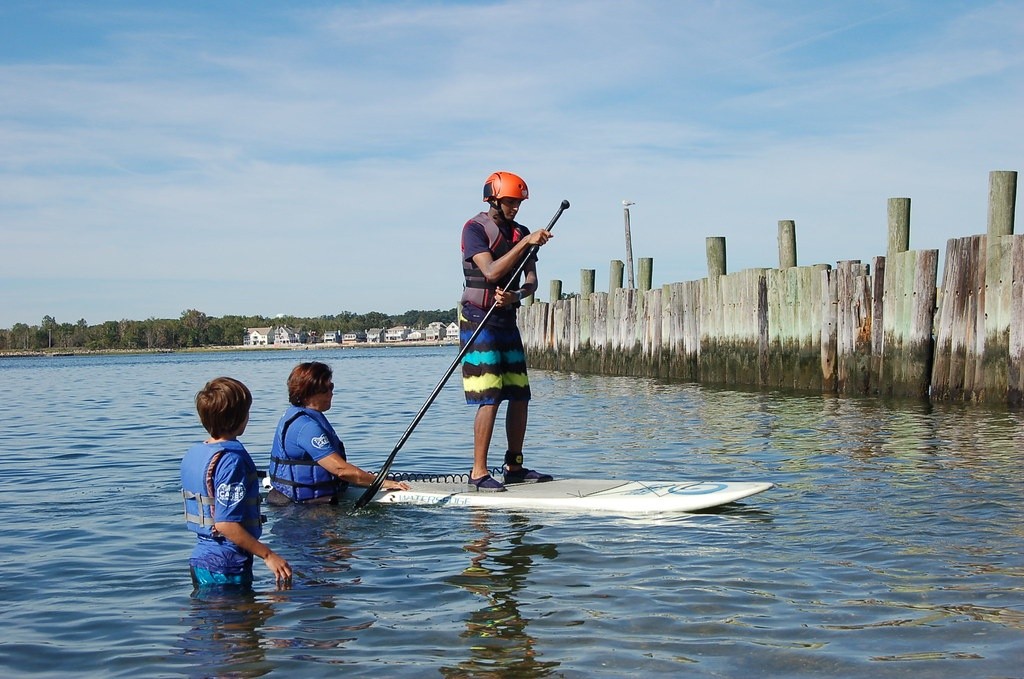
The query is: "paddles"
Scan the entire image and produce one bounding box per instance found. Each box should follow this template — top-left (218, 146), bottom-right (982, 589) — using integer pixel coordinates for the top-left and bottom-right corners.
top-left (348, 199), bottom-right (572, 515)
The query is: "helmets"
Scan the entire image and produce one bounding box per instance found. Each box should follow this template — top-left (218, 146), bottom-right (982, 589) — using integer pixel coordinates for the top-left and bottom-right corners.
top-left (482, 172), bottom-right (528, 203)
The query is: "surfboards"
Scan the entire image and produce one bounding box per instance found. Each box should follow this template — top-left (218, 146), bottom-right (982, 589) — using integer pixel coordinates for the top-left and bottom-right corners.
top-left (347, 467), bottom-right (777, 516)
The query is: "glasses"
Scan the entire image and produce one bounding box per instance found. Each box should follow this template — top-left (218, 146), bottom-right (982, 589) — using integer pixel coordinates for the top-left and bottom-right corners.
top-left (308, 384), bottom-right (334, 393)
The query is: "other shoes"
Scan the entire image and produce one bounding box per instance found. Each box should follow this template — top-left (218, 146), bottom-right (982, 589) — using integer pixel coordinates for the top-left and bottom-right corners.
top-left (467, 471), bottom-right (503, 494)
top-left (503, 466), bottom-right (553, 483)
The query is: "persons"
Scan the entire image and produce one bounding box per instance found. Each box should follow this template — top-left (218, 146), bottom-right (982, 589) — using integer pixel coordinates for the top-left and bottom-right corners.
top-left (180, 377), bottom-right (293, 590)
top-left (269, 362), bottom-right (411, 500)
top-left (459, 172), bottom-right (554, 492)
